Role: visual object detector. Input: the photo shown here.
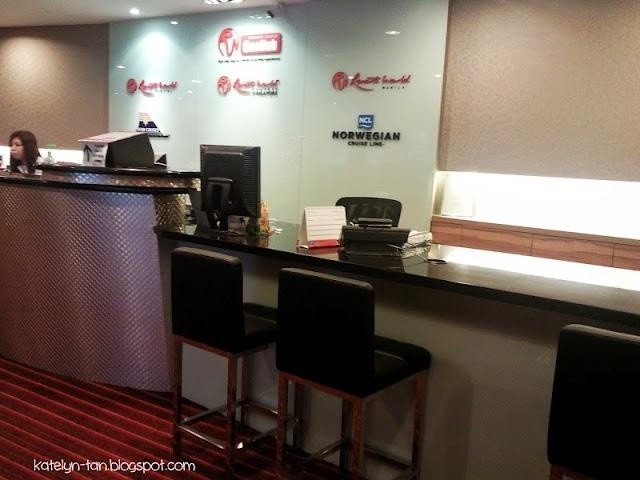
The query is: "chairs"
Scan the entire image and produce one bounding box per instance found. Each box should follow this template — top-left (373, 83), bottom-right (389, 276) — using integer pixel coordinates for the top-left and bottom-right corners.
top-left (334, 193), bottom-right (404, 230)
top-left (166, 244), bottom-right (435, 480)
top-left (544, 320), bottom-right (640, 479)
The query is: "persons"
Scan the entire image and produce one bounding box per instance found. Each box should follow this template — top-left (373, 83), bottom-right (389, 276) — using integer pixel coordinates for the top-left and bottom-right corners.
top-left (8, 130), bottom-right (43, 176)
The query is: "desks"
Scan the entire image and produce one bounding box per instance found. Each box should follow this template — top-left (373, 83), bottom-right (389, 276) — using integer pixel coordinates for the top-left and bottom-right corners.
top-left (151, 216), bottom-right (640, 479)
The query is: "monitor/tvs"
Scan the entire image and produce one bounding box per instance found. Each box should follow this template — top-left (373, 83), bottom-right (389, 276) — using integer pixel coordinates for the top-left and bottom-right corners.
top-left (199, 144), bottom-right (261, 237)
